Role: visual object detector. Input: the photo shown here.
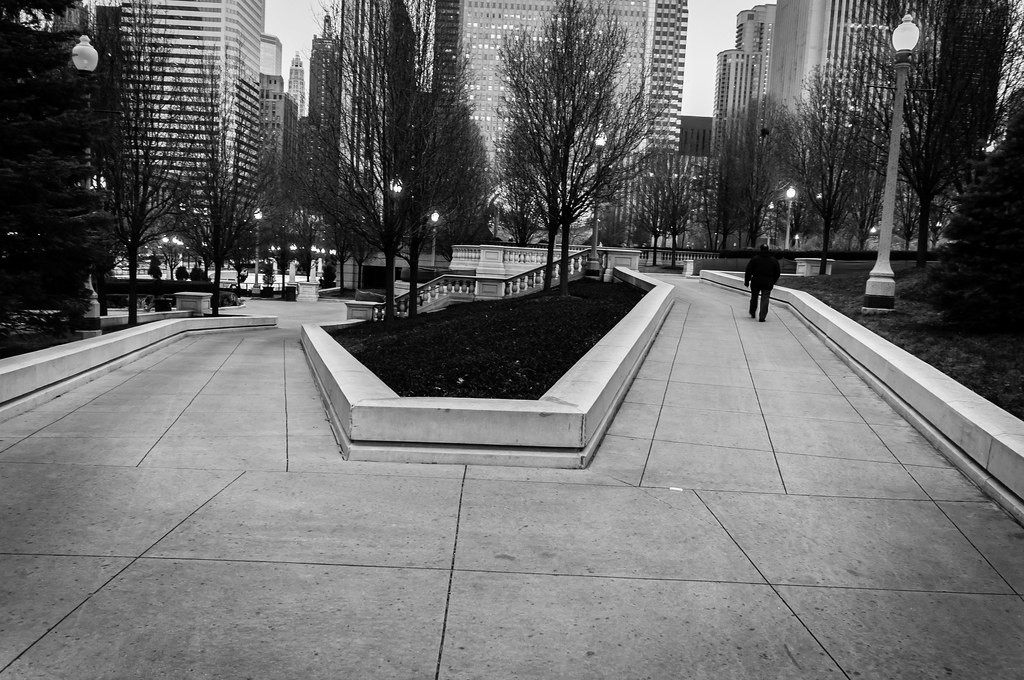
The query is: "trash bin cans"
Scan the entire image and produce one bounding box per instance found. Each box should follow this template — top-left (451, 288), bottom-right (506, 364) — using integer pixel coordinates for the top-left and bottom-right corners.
top-left (153, 298), bottom-right (173, 312)
top-left (285, 286), bottom-right (296, 301)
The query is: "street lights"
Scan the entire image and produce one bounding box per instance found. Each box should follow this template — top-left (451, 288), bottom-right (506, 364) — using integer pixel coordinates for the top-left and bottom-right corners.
top-left (585, 130), bottom-right (607, 280)
top-left (767, 202), bottom-right (774, 248)
top-left (72, 35), bottom-right (104, 340)
top-left (252, 208), bottom-right (261, 298)
top-left (431, 212), bottom-right (439, 268)
top-left (785, 186), bottom-right (796, 250)
top-left (861, 15), bottom-right (919, 313)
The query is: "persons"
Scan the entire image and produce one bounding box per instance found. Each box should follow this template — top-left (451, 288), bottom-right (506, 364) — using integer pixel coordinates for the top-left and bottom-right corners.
top-left (744, 244), bottom-right (781, 322)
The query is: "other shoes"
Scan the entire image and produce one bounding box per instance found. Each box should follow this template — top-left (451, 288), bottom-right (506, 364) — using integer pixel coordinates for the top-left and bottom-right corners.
top-left (759, 315), bottom-right (765, 321)
top-left (751, 312), bottom-right (755, 318)
